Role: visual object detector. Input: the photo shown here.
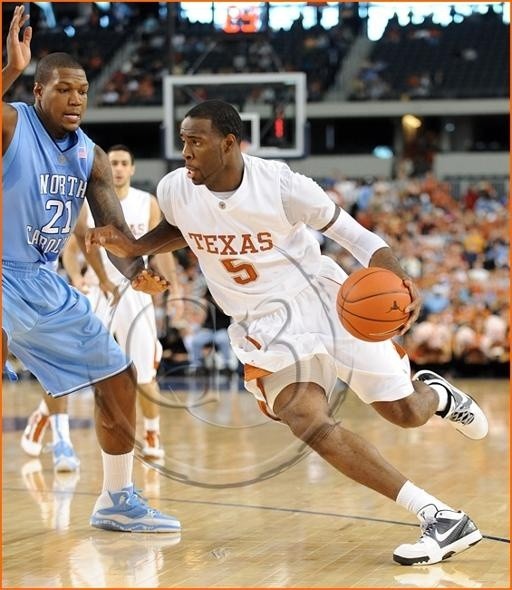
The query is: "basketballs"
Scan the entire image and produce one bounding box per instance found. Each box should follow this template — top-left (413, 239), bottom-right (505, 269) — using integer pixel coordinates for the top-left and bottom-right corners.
top-left (336, 266), bottom-right (411, 341)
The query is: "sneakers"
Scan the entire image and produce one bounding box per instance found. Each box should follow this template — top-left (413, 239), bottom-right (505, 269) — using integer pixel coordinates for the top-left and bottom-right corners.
top-left (89, 483), bottom-right (182, 535)
top-left (412, 369), bottom-right (490, 441)
top-left (142, 428), bottom-right (165, 458)
top-left (20, 408), bottom-right (49, 456)
top-left (393, 503), bottom-right (484, 566)
top-left (51, 439), bottom-right (81, 472)
